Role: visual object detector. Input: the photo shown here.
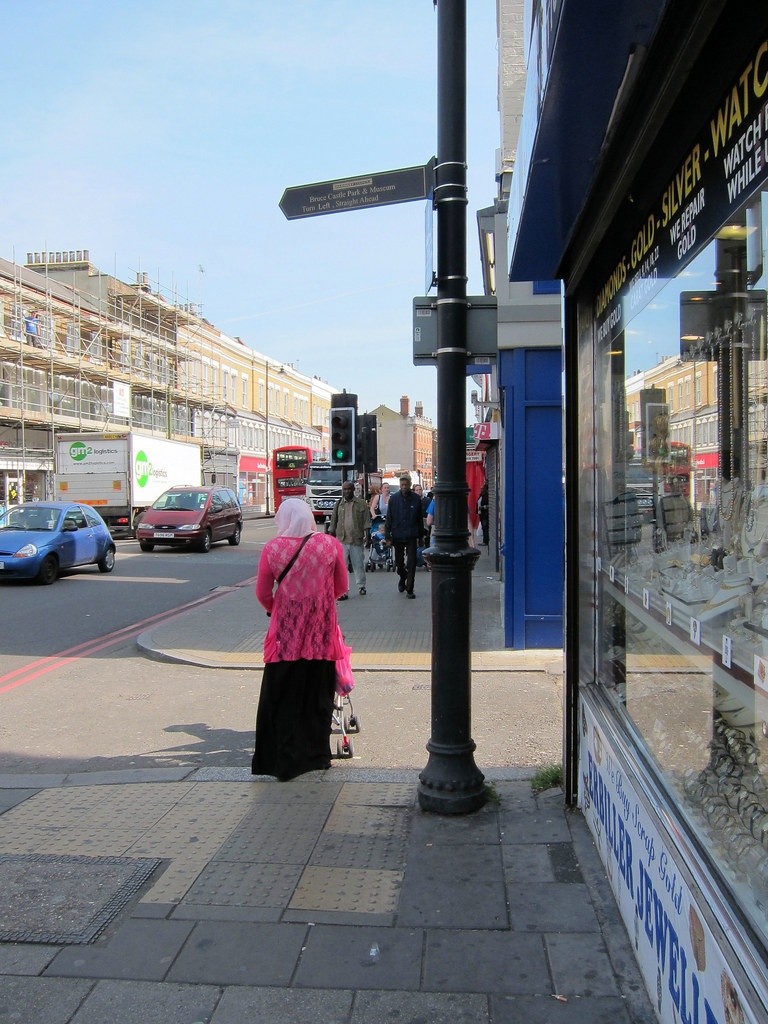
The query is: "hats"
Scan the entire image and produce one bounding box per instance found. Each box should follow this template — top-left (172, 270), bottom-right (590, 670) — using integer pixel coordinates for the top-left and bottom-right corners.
top-left (399, 474), bottom-right (411, 482)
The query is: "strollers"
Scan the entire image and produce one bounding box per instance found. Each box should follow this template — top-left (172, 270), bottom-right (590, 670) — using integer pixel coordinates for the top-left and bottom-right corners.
top-left (332, 690), bottom-right (363, 758)
top-left (365, 513), bottom-right (397, 573)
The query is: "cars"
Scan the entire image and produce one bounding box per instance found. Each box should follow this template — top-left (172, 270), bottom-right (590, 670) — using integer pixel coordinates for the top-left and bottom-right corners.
top-left (0, 501), bottom-right (117, 586)
top-left (136, 485), bottom-right (244, 553)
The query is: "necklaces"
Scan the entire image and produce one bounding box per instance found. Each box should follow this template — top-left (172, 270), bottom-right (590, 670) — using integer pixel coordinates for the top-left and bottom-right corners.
top-left (618, 324), bottom-right (768, 615)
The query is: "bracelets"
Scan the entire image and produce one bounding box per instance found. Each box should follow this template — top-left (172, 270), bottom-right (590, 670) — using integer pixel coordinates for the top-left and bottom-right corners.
top-left (659, 704), bottom-right (768, 872)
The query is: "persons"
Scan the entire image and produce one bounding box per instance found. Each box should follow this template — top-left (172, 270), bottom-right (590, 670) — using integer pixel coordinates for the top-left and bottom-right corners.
top-left (251, 498), bottom-right (348, 781)
top-left (654, 475), bottom-right (694, 552)
top-left (384, 474), bottom-right (423, 599)
top-left (370, 482), bottom-right (489, 572)
top-left (327, 481), bottom-right (372, 601)
top-left (22, 311), bottom-right (40, 347)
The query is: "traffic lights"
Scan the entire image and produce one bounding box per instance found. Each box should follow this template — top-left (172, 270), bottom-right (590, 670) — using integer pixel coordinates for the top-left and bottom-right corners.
top-left (330, 408), bottom-right (353, 465)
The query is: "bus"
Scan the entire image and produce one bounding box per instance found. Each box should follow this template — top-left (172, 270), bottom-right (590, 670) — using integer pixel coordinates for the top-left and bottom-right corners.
top-left (269, 445), bottom-right (332, 523)
top-left (656, 443), bottom-right (695, 505)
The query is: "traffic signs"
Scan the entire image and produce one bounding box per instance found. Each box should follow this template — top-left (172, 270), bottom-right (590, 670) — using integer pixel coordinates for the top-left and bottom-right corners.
top-left (277, 153), bottom-right (438, 221)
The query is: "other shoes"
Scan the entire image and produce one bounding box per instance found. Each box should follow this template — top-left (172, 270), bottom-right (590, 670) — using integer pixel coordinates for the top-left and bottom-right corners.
top-left (337, 593), bottom-right (348, 601)
top-left (359, 587), bottom-right (366, 595)
top-left (406, 593), bottom-right (415, 598)
top-left (398, 575), bottom-right (407, 592)
top-left (382, 555), bottom-right (385, 558)
top-left (478, 542), bottom-right (487, 546)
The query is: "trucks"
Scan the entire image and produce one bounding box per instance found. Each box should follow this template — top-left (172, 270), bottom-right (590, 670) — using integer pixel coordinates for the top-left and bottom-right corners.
top-left (625, 442), bottom-right (661, 521)
top-left (304, 462), bottom-right (383, 525)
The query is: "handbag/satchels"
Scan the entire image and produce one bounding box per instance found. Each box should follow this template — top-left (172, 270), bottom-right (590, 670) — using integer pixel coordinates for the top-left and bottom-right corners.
top-left (370, 495), bottom-right (382, 522)
top-left (334, 625), bottom-right (354, 697)
